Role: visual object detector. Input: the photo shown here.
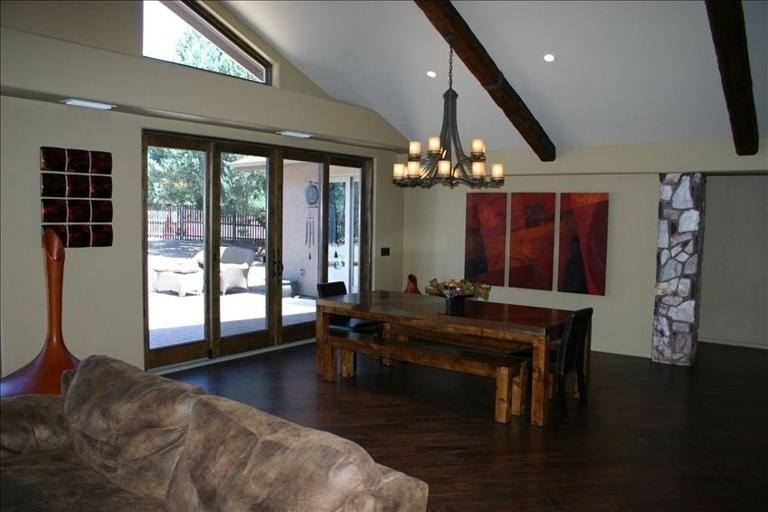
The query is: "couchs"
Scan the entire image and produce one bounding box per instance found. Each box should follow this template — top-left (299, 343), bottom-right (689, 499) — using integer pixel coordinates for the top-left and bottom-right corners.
top-left (0, 368), bottom-right (430, 512)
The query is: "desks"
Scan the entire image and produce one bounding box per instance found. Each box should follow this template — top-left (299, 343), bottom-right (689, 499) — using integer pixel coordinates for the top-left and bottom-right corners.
top-left (153, 267), bottom-right (198, 297)
top-left (315, 291), bottom-right (576, 426)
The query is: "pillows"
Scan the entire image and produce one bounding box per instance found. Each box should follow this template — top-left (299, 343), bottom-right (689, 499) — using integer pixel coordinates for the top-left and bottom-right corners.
top-left (167, 393), bottom-right (380, 512)
top-left (63, 354), bottom-right (204, 501)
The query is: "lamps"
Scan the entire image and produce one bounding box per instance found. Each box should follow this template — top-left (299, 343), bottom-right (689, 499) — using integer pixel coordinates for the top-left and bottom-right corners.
top-left (392, 33), bottom-right (505, 191)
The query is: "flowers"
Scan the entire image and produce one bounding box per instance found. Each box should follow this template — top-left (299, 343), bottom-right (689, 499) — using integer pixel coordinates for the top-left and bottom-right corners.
top-left (424, 278), bottom-right (493, 300)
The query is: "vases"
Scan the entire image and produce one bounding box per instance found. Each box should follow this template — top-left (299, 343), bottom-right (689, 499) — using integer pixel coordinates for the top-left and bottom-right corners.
top-left (0, 228), bottom-right (82, 398)
top-left (404, 272), bottom-right (421, 296)
top-left (445, 298), bottom-right (466, 316)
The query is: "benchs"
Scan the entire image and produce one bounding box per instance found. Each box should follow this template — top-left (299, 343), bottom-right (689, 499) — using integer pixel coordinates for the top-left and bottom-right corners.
top-left (321, 330), bottom-right (528, 424)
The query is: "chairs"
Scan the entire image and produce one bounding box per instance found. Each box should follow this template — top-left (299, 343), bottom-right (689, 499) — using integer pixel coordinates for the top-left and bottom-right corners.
top-left (192, 246), bottom-right (256, 296)
top-left (504, 307), bottom-right (594, 422)
top-left (316, 281), bottom-right (384, 376)
